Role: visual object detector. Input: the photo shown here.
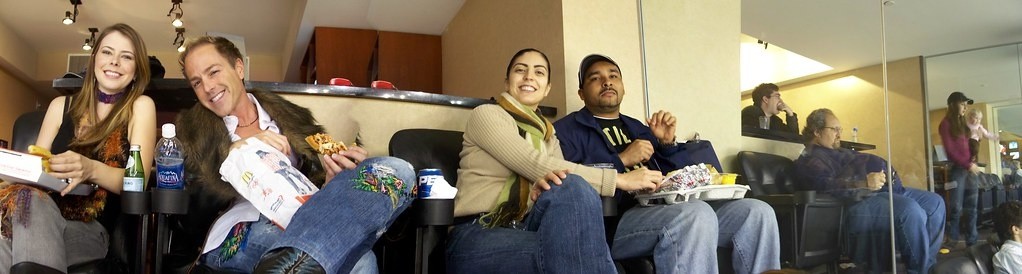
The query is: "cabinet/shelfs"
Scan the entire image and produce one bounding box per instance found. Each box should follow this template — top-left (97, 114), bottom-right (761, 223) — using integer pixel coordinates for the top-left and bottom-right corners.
top-left (300, 27), bottom-right (377, 89)
top-left (367, 30), bottom-right (443, 95)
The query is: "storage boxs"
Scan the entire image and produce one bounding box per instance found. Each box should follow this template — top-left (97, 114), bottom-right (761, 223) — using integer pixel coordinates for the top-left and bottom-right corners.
top-left (0, 147), bottom-right (91, 195)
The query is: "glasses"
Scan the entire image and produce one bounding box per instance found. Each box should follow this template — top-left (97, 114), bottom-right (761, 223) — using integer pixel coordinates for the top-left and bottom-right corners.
top-left (770, 95), bottom-right (780, 98)
top-left (823, 127), bottom-right (844, 132)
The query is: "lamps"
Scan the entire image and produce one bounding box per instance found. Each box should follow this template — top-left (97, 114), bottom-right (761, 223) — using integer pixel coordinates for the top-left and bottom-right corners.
top-left (167, 0), bottom-right (183, 27)
top-left (82, 28), bottom-right (99, 50)
top-left (172, 28), bottom-right (185, 52)
top-left (62, 0), bottom-right (82, 25)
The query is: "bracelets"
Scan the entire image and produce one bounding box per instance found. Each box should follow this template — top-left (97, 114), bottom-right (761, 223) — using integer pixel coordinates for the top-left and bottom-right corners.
top-left (660, 139), bottom-right (674, 147)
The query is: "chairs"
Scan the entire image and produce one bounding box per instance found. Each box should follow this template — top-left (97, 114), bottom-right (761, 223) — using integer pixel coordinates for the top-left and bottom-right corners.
top-left (388, 127), bottom-right (905, 274)
top-left (11, 110), bottom-right (388, 274)
top-left (929, 172), bottom-right (1022, 274)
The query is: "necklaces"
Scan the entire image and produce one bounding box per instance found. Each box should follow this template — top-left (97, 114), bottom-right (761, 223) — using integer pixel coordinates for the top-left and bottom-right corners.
top-left (237, 116), bottom-right (258, 128)
top-left (96, 88), bottom-right (126, 104)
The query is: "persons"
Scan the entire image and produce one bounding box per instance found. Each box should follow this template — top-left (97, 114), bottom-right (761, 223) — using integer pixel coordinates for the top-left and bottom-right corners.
top-left (0, 23), bottom-right (156, 274)
top-left (741, 82), bottom-right (799, 140)
top-left (441, 48), bottom-right (663, 274)
top-left (992, 200), bottom-right (1022, 274)
top-left (171, 35), bottom-right (416, 274)
top-left (938, 92), bottom-right (982, 253)
top-left (1009, 160), bottom-right (1022, 188)
top-left (552, 53), bottom-right (780, 274)
top-left (965, 106), bottom-right (998, 164)
top-left (789, 107), bottom-right (946, 274)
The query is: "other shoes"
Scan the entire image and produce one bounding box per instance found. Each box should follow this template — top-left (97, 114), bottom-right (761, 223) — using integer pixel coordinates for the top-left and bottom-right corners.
top-left (941, 240), bottom-right (958, 249)
top-left (967, 243), bottom-right (974, 247)
top-left (254, 246), bottom-right (326, 274)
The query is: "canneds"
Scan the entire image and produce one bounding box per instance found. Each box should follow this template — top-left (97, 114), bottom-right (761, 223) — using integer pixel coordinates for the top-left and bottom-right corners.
top-left (417, 169), bottom-right (443, 198)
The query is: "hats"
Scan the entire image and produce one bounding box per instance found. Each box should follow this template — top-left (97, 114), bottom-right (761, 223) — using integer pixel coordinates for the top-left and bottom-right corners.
top-left (578, 53), bottom-right (621, 85)
top-left (948, 92), bottom-right (974, 105)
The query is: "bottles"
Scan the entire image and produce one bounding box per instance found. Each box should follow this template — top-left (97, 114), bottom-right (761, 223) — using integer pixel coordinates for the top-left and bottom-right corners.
top-left (122, 145), bottom-right (146, 191)
top-left (852, 128), bottom-right (858, 143)
top-left (153, 123), bottom-right (184, 189)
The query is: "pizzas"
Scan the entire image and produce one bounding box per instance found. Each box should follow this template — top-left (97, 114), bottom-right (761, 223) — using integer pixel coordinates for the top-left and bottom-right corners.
top-left (304, 133), bottom-right (348, 155)
top-left (26, 145), bottom-right (53, 172)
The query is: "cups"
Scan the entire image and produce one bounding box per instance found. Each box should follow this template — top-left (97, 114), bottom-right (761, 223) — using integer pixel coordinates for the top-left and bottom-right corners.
top-left (759, 115), bottom-right (771, 130)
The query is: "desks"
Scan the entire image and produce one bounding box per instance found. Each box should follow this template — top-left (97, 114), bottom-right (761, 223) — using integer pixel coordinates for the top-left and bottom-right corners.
top-left (932, 165), bottom-right (954, 222)
top-left (52, 78), bottom-right (497, 108)
top-left (742, 127), bottom-right (876, 151)
